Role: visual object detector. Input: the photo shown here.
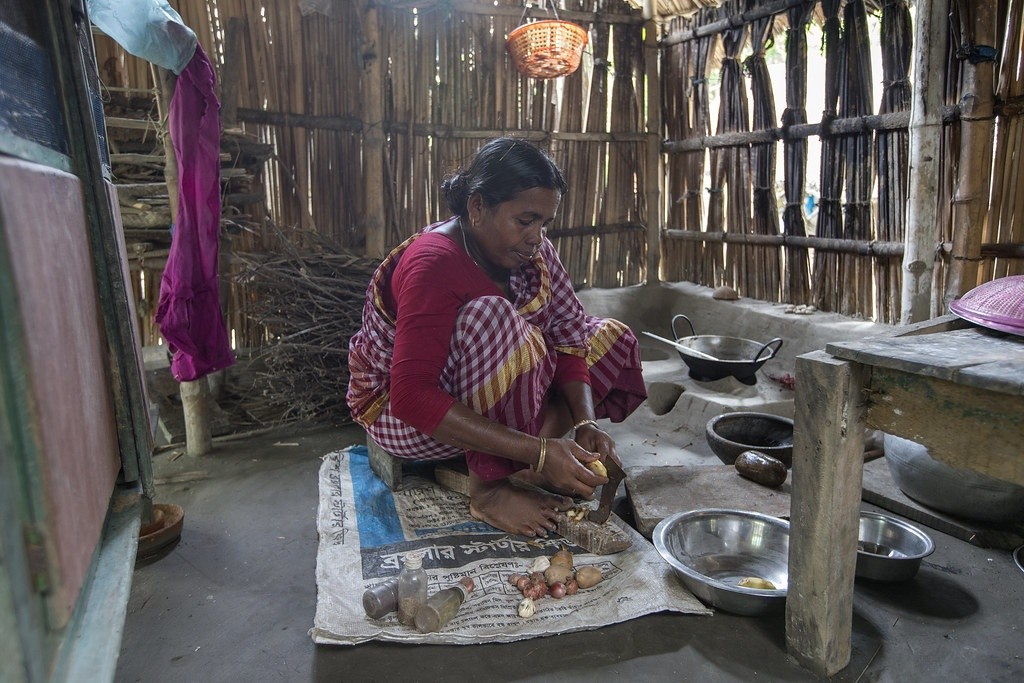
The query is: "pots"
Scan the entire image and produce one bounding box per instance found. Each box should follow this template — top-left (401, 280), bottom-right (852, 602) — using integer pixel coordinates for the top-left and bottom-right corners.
top-left (669, 313), bottom-right (783, 384)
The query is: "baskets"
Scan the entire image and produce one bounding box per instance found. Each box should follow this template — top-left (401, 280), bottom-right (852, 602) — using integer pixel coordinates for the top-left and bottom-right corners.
top-left (504, 20), bottom-right (588, 80)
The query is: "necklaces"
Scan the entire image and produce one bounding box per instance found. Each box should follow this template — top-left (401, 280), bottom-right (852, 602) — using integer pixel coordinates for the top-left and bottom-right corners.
top-left (458, 217), bottom-right (478, 267)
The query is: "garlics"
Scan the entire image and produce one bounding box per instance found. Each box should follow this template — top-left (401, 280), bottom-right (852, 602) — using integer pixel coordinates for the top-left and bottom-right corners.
top-left (518, 597), bottom-right (536, 618)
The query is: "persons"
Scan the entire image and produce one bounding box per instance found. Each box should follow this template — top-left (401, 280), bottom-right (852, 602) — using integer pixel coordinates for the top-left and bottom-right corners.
top-left (348, 137), bottom-right (647, 540)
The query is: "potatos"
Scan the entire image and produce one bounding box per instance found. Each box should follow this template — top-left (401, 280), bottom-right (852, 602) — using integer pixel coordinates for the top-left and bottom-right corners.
top-left (545, 550), bottom-right (602, 588)
top-left (739, 577), bottom-right (777, 589)
top-left (586, 458), bottom-right (608, 477)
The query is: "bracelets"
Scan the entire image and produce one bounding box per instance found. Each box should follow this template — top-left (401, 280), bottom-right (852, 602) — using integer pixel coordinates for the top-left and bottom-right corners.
top-left (574, 419), bottom-right (599, 430)
top-left (533, 436), bottom-right (546, 473)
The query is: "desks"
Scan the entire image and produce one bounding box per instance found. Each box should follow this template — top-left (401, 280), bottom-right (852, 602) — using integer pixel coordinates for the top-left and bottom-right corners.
top-left (778, 311), bottom-right (1024, 675)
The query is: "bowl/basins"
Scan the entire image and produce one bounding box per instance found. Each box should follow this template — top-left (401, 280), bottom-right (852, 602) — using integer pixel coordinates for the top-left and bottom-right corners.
top-left (851, 508), bottom-right (936, 584)
top-left (705, 412), bottom-right (795, 472)
top-left (651, 508), bottom-right (797, 618)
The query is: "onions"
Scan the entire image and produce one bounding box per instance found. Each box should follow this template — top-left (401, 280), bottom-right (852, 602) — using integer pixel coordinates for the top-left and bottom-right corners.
top-left (509, 572), bottom-right (579, 600)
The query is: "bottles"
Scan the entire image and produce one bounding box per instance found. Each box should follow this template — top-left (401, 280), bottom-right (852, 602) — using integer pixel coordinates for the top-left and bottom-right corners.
top-left (414, 585), bottom-right (467, 633)
top-left (363, 577), bottom-right (398, 618)
top-left (398, 552), bottom-right (428, 625)
